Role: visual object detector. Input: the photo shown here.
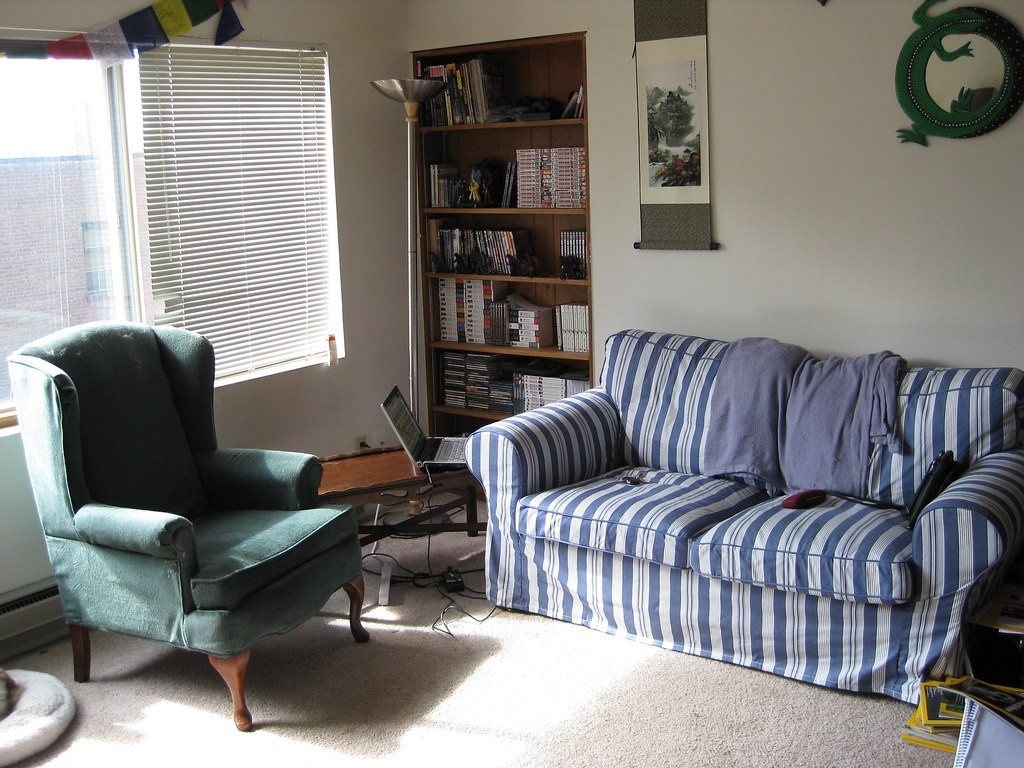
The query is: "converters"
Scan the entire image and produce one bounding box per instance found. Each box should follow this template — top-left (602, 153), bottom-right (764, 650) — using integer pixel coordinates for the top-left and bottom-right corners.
top-left (442, 570), bottom-right (465, 594)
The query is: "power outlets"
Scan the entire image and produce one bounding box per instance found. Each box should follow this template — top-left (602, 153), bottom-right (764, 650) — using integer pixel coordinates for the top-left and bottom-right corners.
top-left (354, 434), bottom-right (367, 451)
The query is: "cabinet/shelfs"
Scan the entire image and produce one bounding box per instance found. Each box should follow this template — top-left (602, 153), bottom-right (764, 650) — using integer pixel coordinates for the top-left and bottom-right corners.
top-left (409, 30), bottom-right (592, 437)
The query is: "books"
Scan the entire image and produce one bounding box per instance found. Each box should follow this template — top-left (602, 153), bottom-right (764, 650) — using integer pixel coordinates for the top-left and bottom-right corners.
top-left (438, 350), bottom-right (590, 415)
top-left (426, 218), bottom-right (534, 276)
top-left (900, 675), bottom-right (1024, 768)
top-left (429, 277), bottom-right (589, 352)
top-left (559, 82), bottom-right (585, 119)
top-left (428, 147), bottom-right (586, 209)
top-left (560, 229), bottom-right (588, 279)
top-left (416, 58), bottom-right (500, 126)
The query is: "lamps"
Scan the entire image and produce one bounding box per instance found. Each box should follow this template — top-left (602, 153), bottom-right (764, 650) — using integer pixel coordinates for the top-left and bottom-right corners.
top-left (370, 78), bottom-right (450, 540)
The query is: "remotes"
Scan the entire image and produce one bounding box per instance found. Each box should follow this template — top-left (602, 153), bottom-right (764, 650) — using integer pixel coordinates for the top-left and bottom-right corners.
top-left (622, 477), bottom-right (640, 485)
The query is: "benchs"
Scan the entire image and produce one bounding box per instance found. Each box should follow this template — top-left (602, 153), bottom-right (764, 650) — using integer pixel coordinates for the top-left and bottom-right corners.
top-left (316, 445), bottom-right (480, 538)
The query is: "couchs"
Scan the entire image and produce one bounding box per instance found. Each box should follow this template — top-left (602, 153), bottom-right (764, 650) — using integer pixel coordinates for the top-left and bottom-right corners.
top-left (463, 328), bottom-right (1024, 704)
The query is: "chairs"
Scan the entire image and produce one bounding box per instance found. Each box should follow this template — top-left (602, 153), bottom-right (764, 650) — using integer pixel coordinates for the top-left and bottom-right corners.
top-left (6, 320), bottom-right (371, 732)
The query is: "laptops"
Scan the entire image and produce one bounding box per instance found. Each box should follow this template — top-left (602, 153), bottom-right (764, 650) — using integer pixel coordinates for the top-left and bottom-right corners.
top-left (381, 384), bottom-right (468, 472)
